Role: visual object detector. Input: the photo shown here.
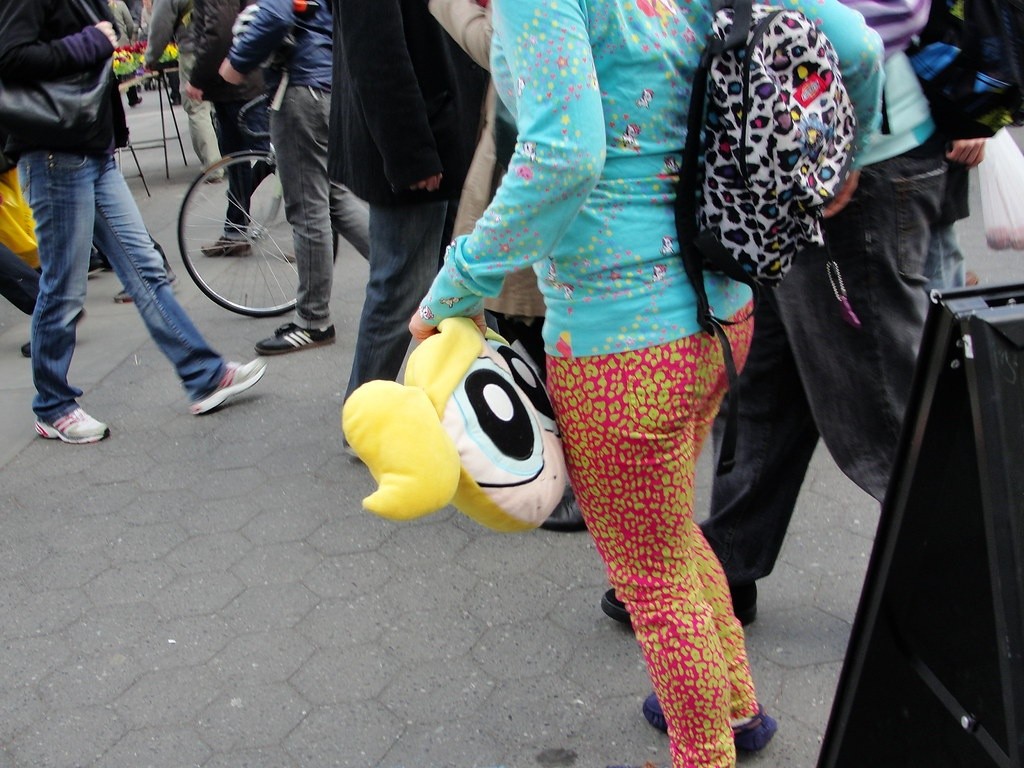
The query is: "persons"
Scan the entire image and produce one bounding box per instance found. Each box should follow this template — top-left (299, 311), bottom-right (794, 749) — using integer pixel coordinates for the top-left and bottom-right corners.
top-left (410, 0), bottom-right (888, 768)
top-left (0, 0), bottom-right (1024, 627)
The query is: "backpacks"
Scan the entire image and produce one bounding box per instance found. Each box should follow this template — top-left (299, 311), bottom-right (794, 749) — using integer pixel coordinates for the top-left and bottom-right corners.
top-left (675, 0), bottom-right (862, 478)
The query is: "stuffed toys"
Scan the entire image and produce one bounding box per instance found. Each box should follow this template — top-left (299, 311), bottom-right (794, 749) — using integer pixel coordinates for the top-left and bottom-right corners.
top-left (341, 318), bottom-right (570, 537)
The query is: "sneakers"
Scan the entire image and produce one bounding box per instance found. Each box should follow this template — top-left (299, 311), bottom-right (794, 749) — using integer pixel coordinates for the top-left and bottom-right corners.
top-left (188, 357), bottom-right (269, 415)
top-left (254, 322), bottom-right (337, 356)
top-left (34, 406), bottom-right (124, 447)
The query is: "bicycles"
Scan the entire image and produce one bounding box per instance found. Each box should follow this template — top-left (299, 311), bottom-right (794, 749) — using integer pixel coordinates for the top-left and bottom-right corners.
top-left (175, 89), bottom-right (341, 319)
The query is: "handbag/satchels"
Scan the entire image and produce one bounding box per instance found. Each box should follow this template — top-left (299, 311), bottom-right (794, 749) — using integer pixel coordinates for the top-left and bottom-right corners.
top-left (982, 129), bottom-right (1023, 253)
top-left (0, 56), bottom-right (120, 159)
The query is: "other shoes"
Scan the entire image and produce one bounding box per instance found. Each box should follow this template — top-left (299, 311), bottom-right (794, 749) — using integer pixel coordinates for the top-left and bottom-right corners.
top-left (642, 687), bottom-right (779, 751)
top-left (113, 269), bottom-right (177, 303)
top-left (537, 485), bottom-right (588, 533)
top-left (599, 574), bottom-right (759, 632)
top-left (22, 308), bottom-right (88, 357)
top-left (201, 236), bottom-right (262, 263)
